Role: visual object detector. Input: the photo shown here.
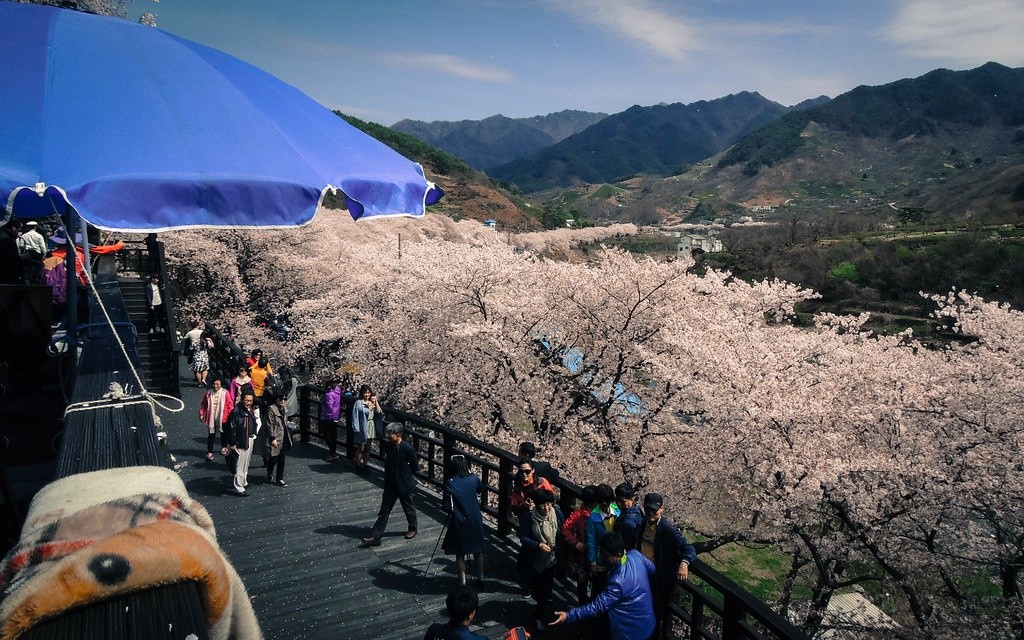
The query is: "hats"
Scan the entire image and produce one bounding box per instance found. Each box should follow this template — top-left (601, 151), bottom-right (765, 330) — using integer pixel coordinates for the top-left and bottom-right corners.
top-left (643, 492), bottom-right (663, 510)
top-left (26, 220), bottom-right (38, 228)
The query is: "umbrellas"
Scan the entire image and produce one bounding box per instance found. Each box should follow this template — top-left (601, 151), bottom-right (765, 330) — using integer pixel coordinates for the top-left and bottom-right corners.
top-left (0, 0), bottom-right (445, 395)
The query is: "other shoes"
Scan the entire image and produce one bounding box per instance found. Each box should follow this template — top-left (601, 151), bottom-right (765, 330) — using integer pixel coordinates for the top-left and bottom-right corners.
top-left (267, 471), bottom-right (273, 481)
top-left (239, 490), bottom-right (249, 496)
top-left (207, 453), bottom-right (214, 460)
top-left (326, 454), bottom-right (338, 461)
top-left (244, 483), bottom-right (253, 489)
top-left (276, 479), bottom-right (287, 487)
top-left (202, 380), bottom-right (207, 386)
top-left (522, 587), bottom-right (531, 599)
top-left (355, 459), bottom-right (365, 466)
top-left (221, 448), bottom-right (229, 455)
top-left (547, 592), bottom-right (554, 601)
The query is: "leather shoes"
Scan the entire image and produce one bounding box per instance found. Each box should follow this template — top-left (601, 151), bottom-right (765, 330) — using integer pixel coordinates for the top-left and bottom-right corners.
top-left (405, 529), bottom-right (416, 538)
top-left (362, 536), bottom-right (382, 546)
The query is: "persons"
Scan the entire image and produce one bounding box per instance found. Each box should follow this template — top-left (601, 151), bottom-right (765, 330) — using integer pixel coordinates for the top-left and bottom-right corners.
top-left (176, 320), bottom-right (214, 388)
top-left (423, 588), bottom-right (488, 640)
top-left (363, 422), bottom-right (418, 546)
top-left (442, 455), bottom-right (487, 587)
top-left (143, 233), bottom-right (157, 279)
top-left (0, 216), bottom-right (87, 285)
top-left (145, 273), bottom-right (166, 334)
top-left (199, 349), bottom-right (298, 496)
top-left (352, 385), bottom-right (383, 473)
top-left (548, 532), bottom-right (656, 640)
top-left (270, 320), bottom-right (282, 333)
top-left (510, 442), bottom-right (695, 640)
top-left (320, 380), bottom-right (341, 460)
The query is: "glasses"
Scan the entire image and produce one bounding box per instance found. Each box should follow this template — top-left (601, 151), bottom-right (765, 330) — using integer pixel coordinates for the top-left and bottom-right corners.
top-left (597, 547), bottom-right (614, 557)
top-left (518, 469), bottom-right (533, 474)
top-left (277, 398), bottom-right (288, 402)
top-left (239, 370), bottom-right (247, 373)
